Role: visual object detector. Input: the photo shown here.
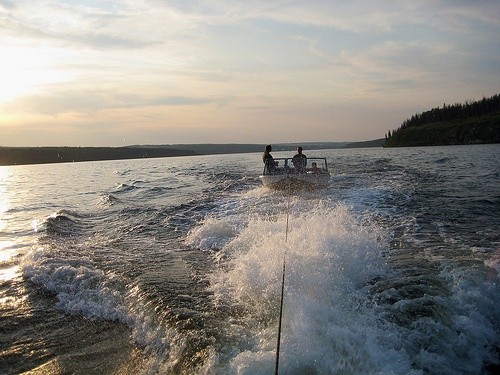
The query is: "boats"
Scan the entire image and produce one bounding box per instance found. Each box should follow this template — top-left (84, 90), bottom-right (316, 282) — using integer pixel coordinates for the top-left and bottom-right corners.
top-left (261, 157), bottom-right (331, 193)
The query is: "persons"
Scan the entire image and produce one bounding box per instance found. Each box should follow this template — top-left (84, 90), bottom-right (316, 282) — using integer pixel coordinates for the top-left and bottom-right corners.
top-left (311, 162), bottom-right (322, 174)
top-left (262, 145), bottom-right (279, 172)
top-left (291, 146), bottom-right (308, 174)
